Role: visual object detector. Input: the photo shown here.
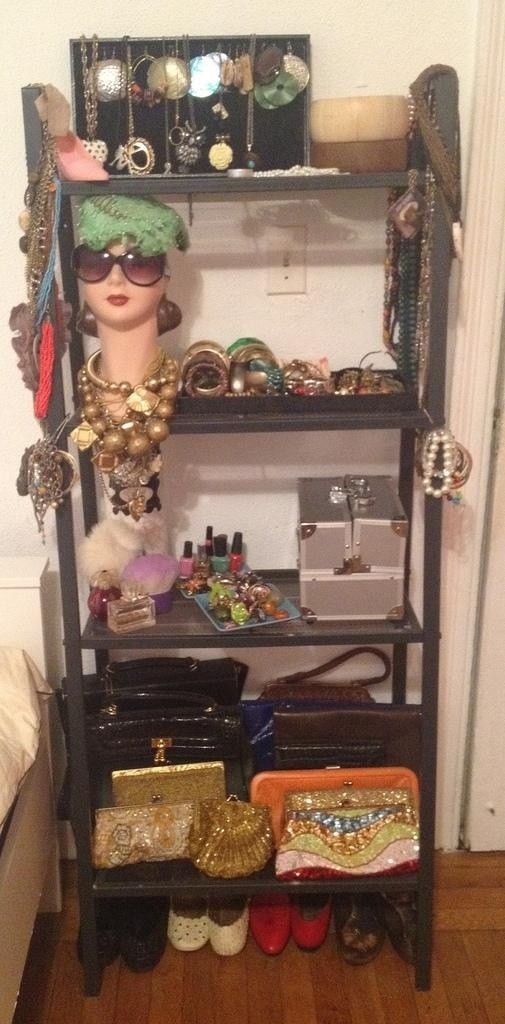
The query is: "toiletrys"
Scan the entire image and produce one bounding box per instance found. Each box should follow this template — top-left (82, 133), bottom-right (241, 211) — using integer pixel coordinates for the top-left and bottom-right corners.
top-left (84, 524), bottom-right (244, 630)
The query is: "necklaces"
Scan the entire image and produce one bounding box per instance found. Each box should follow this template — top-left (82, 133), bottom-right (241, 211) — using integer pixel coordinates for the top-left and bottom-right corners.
top-left (77, 352), bottom-right (181, 521)
top-left (14, 85), bottom-right (66, 421)
top-left (78, 34), bottom-right (337, 178)
top-left (381, 67), bottom-right (462, 405)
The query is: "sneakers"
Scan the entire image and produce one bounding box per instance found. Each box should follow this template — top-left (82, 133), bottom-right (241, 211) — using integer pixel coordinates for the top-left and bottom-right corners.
top-left (381, 892), bottom-right (420, 966)
top-left (121, 897), bottom-right (166, 975)
top-left (77, 898), bottom-right (123, 969)
top-left (336, 893), bottom-right (387, 966)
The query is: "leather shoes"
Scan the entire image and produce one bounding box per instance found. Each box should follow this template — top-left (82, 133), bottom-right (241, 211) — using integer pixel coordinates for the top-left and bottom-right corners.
top-left (250, 894), bottom-right (292, 957)
top-left (289, 894), bottom-right (331, 952)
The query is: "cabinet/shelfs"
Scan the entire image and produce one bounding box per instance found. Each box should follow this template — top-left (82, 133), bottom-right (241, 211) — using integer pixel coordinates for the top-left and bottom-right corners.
top-left (19, 64), bottom-right (460, 997)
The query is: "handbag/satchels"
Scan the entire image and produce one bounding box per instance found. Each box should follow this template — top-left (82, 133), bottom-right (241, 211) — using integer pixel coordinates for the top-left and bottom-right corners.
top-left (56, 645), bottom-right (427, 883)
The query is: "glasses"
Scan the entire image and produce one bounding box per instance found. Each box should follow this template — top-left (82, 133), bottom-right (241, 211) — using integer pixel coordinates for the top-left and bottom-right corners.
top-left (70, 241), bottom-right (171, 289)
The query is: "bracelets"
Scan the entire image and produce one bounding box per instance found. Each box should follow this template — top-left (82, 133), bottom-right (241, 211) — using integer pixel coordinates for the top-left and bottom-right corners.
top-left (179, 337), bottom-right (405, 397)
top-left (18, 443), bottom-right (79, 547)
top-left (415, 426), bottom-right (471, 507)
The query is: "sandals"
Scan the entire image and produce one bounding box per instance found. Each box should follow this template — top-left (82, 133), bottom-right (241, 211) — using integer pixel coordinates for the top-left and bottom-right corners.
top-left (207, 891), bottom-right (249, 958)
top-left (166, 895), bottom-right (208, 953)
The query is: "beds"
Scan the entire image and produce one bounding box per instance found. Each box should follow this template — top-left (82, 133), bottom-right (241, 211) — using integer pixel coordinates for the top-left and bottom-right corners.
top-left (0, 554), bottom-right (64, 1024)
